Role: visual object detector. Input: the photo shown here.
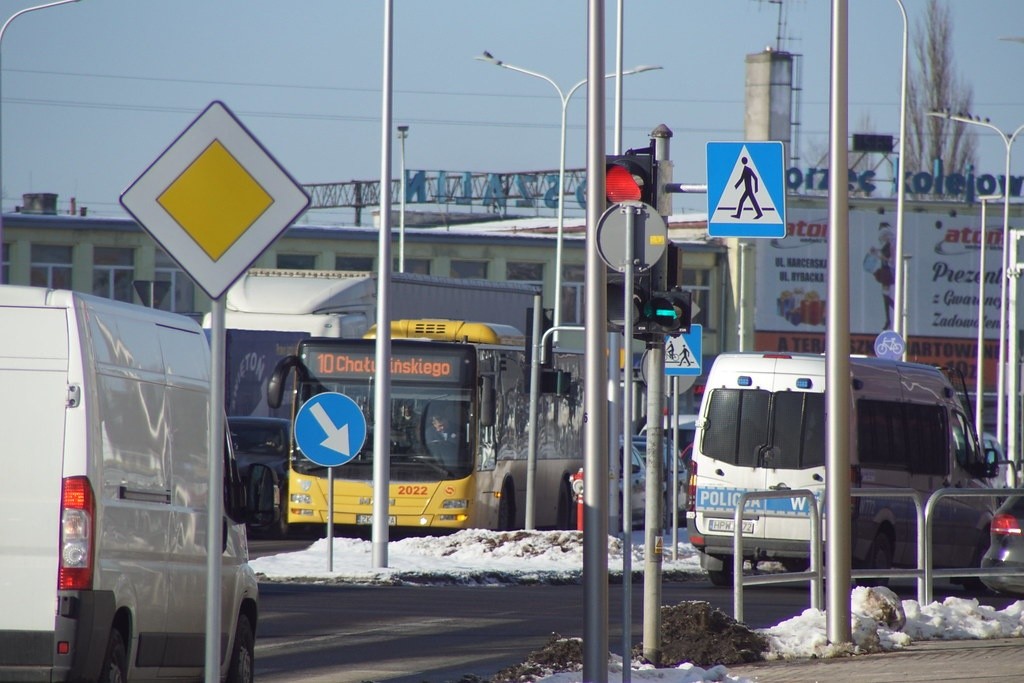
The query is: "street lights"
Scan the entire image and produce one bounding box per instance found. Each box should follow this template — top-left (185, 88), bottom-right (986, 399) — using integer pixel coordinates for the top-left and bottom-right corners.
top-left (473, 50), bottom-right (664, 344)
top-left (927, 105), bottom-right (1023, 449)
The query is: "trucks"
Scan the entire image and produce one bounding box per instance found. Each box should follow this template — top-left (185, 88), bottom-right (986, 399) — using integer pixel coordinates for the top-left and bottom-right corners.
top-left (202, 270), bottom-right (548, 532)
top-left (2, 282), bottom-right (259, 683)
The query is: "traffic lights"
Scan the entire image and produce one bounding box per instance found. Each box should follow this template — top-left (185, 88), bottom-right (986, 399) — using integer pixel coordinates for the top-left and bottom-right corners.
top-left (642, 288), bottom-right (690, 333)
top-left (601, 148), bottom-right (657, 209)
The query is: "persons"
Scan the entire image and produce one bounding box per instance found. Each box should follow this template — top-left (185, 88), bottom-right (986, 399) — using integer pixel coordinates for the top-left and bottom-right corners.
top-left (396, 399), bottom-right (421, 431)
top-left (426, 414), bottom-right (465, 446)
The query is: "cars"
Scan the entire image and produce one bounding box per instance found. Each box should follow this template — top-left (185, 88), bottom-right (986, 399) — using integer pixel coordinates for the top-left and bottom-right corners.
top-left (616, 433), bottom-right (690, 535)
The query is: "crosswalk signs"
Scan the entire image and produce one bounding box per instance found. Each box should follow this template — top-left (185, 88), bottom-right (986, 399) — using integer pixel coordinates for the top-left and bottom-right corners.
top-left (704, 139), bottom-right (787, 239)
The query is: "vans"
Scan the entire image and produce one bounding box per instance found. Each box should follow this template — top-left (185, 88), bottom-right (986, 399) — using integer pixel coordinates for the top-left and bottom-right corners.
top-left (689, 345), bottom-right (1004, 582)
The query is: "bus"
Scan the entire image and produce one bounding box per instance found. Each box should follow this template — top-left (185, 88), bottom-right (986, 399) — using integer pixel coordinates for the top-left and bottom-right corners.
top-left (258, 315), bottom-right (585, 536)
